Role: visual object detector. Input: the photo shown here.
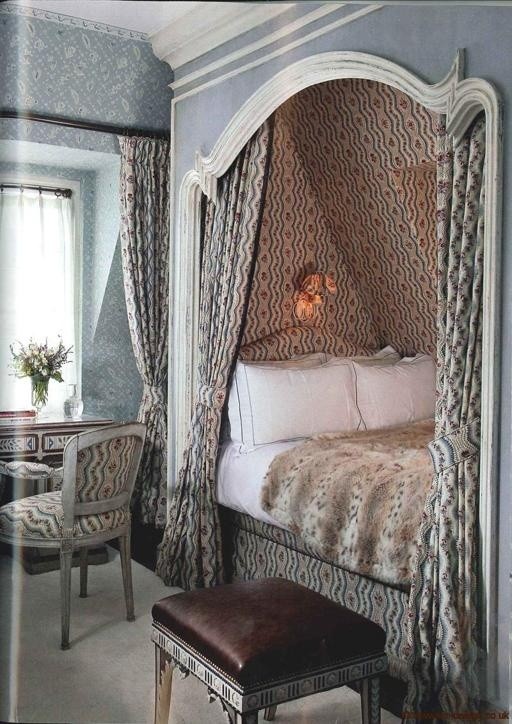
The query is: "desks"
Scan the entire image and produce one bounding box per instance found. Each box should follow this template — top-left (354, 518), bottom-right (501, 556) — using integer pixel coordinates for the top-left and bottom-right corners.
top-left (0, 411), bottom-right (117, 574)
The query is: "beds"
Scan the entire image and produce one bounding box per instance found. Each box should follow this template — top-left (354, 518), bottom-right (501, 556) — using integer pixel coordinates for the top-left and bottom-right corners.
top-left (153, 2), bottom-right (511, 721)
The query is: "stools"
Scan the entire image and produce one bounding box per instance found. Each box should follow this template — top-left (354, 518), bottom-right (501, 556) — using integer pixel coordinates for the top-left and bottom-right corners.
top-left (150, 578), bottom-right (386, 723)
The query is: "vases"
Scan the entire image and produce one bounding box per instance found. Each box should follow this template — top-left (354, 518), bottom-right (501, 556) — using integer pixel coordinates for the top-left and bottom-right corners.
top-left (30, 380), bottom-right (50, 419)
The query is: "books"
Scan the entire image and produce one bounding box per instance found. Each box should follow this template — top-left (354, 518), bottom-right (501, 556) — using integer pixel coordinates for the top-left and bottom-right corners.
top-left (0, 409), bottom-right (38, 417)
top-left (0, 417), bottom-right (37, 424)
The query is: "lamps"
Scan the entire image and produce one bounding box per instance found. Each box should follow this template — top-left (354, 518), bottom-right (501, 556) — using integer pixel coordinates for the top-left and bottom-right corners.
top-left (292, 271), bottom-right (337, 323)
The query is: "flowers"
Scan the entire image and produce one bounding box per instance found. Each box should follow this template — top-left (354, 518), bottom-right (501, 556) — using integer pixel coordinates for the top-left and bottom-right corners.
top-left (8, 334), bottom-right (74, 406)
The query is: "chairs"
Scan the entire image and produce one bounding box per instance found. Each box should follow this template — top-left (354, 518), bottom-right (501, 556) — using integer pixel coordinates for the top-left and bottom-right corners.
top-left (0, 421), bottom-right (148, 649)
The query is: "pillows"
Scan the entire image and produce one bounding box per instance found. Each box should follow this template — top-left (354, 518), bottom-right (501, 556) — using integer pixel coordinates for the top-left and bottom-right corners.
top-left (227, 345), bottom-right (435, 458)
